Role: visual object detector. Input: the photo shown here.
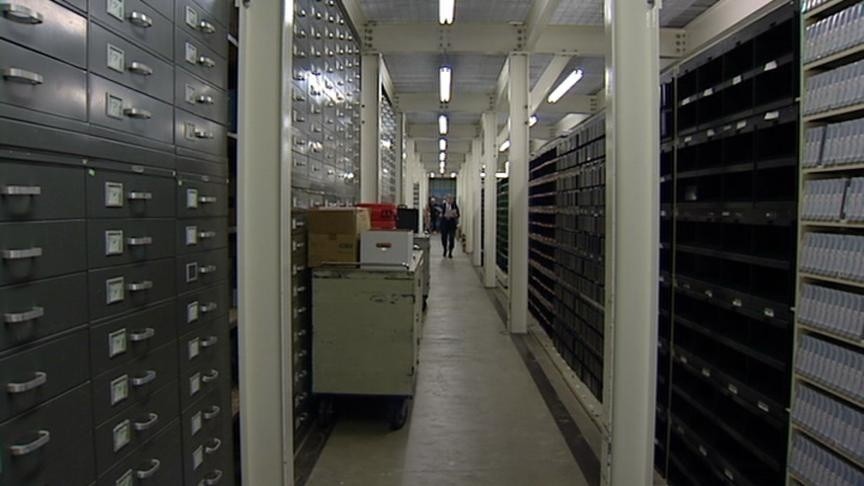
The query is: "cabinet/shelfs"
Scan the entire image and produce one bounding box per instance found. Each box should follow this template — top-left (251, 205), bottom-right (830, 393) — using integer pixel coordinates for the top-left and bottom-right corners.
top-left (497, 1), bottom-right (859, 485)
top-left (1, 1), bottom-right (421, 484)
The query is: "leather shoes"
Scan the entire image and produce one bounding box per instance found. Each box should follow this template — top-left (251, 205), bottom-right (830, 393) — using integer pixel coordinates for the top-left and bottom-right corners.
top-left (442, 246), bottom-right (453, 258)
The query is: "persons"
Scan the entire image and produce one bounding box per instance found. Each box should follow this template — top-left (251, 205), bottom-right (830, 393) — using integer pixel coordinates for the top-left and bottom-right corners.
top-left (437, 192), bottom-right (461, 260)
top-left (428, 194), bottom-right (440, 234)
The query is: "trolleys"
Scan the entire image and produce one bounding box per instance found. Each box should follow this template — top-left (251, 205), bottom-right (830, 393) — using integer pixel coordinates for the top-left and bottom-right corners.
top-left (309, 231), bottom-right (431, 432)
